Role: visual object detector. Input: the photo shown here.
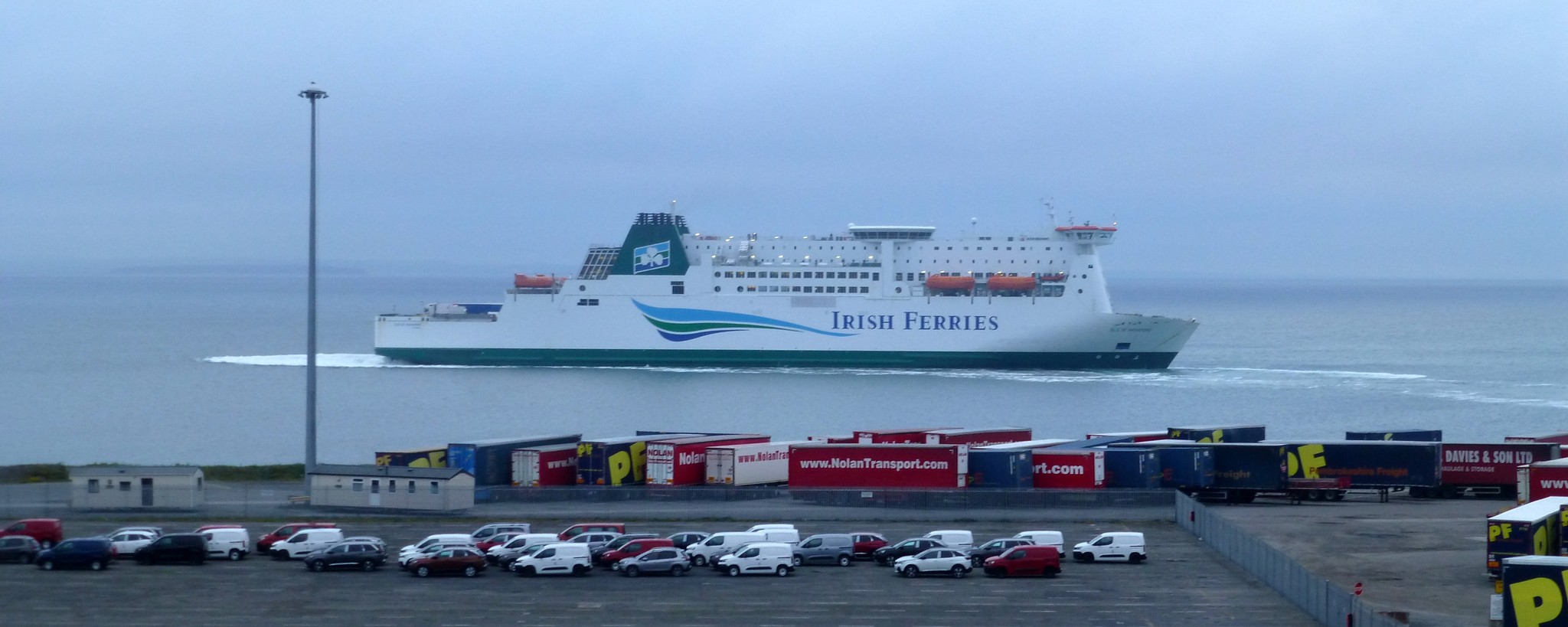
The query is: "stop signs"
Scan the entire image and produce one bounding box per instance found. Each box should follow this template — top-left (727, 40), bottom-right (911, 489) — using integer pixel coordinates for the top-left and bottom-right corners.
top-left (1354, 582), bottom-right (1363, 595)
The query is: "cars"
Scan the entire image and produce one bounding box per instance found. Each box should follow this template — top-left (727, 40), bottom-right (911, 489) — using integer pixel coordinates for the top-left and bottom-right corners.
top-left (893, 547), bottom-right (974, 579)
top-left (104, 526), bottom-right (162, 561)
top-left (0, 535), bottom-right (42, 565)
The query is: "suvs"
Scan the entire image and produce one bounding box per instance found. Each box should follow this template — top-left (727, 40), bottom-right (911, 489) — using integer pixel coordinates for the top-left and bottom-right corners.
top-left (407, 546), bottom-right (489, 579)
top-left (394, 519), bottom-right (1065, 568)
top-left (302, 535), bottom-right (388, 573)
top-left (35, 537), bottom-right (118, 572)
top-left (618, 546), bottom-right (692, 578)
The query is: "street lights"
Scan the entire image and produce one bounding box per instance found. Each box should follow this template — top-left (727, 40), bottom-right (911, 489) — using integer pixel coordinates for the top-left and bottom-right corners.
top-left (1041, 270), bottom-right (1064, 281)
top-left (371, 193), bottom-right (1201, 371)
top-left (298, 78), bottom-right (330, 492)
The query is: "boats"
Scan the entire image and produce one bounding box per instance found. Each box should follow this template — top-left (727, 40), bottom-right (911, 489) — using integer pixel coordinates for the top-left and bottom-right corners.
top-left (514, 272), bottom-right (558, 288)
top-left (987, 271), bottom-right (1037, 290)
top-left (925, 270), bottom-right (975, 291)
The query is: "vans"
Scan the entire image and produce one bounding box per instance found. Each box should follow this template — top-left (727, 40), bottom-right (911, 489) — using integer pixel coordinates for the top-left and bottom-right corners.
top-left (1072, 531), bottom-right (1146, 565)
top-left (0, 517), bottom-right (64, 550)
top-left (508, 541), bottom-right (594, 578)
top-left (982, 544), bottom-right (1062, 578)
top-left (193, 524), bottom-right (251, 561)
top-left (256, 521), bottom-right (337, 553)
top-left (268, 527), bottom-right (344, 561)
top-left (133, 532), bottom-right (209, 566)
top-left (718, 542), bottom-right (796, 577)
